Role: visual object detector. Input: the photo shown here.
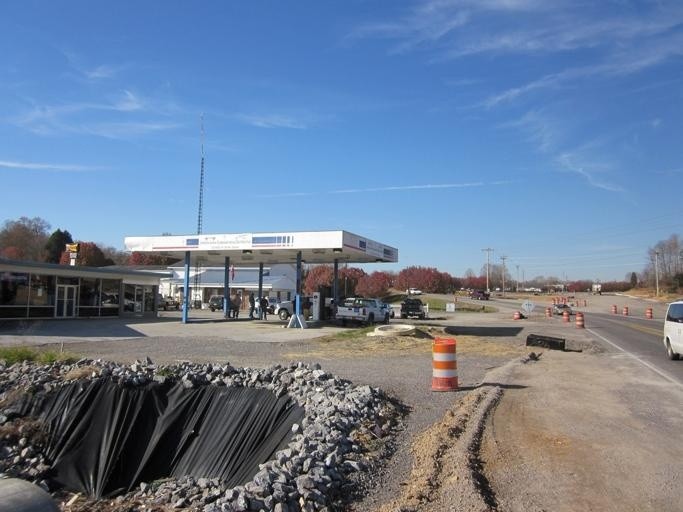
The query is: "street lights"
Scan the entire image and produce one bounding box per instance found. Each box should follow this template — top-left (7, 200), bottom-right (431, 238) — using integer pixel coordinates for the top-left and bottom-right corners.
top-left (652, 249), bottom-right (659, 296)
top-left (482, 247), bottom-right (494, 293)
top-left (513, 265), bottom-right (520, 292)
top-left (499, 255), bottom-right (509, 296)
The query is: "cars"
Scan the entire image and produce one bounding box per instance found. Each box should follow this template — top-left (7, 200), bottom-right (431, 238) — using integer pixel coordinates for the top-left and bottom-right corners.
top-left (163, 296), bottom-right (180, 310)
top-left (104, 292), bottom-right (139, 311)
top-left (385, 303), bottom-right (395, 318)
top-left (405, 288), bottom-right (422, 295)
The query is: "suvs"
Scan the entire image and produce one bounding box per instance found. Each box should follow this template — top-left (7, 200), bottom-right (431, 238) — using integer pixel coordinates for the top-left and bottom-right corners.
top-left (144, 293), bottom-right (169, 311)
top-left (273, 297), bottom-right (334, 320)
top-left (207, 295), bottom-right (237, 312)
top-left (336, 298), bottom-right (390, 326)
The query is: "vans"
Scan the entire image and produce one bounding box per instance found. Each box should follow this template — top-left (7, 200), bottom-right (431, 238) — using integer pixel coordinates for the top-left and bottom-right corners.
top-left (251, 296), bottom-right (281, 315)
top-left (661, 299), bottom-right (683, 361)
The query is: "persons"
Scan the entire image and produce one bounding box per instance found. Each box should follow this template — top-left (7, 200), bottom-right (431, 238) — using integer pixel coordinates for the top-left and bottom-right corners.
top-left (260, 296), bottom-right (268, 320)
top-left (233, 294), bottom-right (241, 317)
top-left (249, 293), bottom-right (254, 318)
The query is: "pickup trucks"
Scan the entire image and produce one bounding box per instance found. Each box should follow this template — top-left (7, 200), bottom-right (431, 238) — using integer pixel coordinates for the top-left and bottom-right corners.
top-left (399, 299), bottom-right (425, 319)
top-left (469, 291), bottom-right (489, 302)
